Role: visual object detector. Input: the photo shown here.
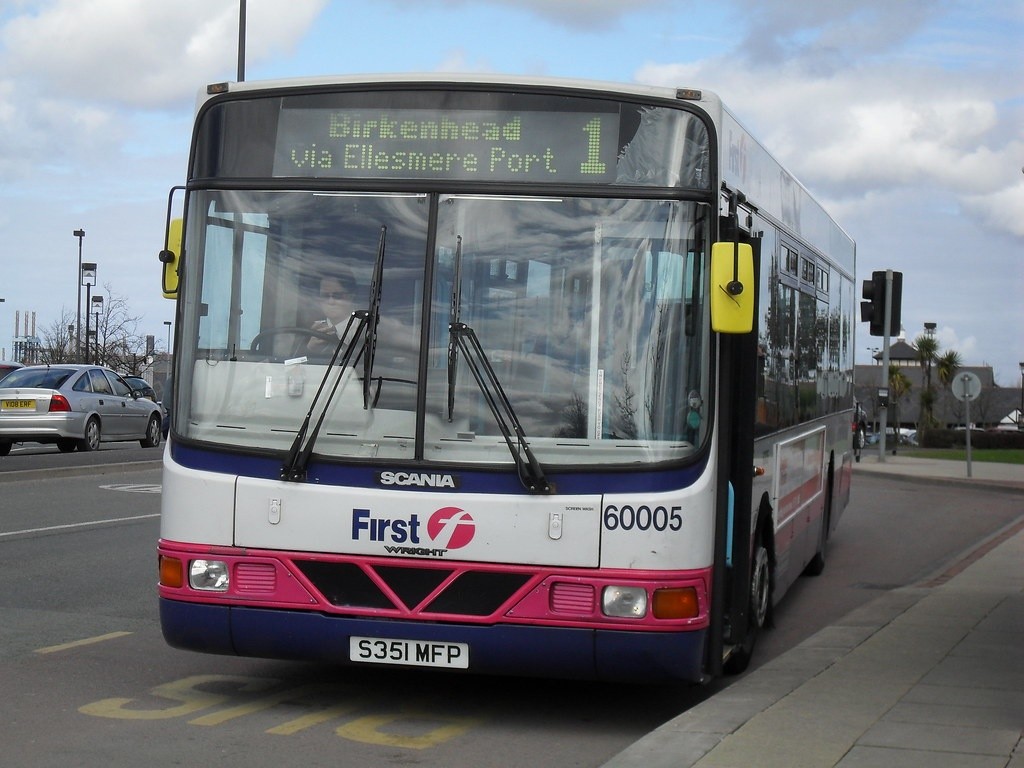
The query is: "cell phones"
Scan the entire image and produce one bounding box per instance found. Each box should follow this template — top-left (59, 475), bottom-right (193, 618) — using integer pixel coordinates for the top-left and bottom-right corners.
top-left (315, 320), bottom-right (329, 329)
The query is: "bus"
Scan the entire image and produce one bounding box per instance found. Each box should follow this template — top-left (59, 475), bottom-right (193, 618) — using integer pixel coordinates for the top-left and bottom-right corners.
top-left (154, 72), bottom-right (860, 692)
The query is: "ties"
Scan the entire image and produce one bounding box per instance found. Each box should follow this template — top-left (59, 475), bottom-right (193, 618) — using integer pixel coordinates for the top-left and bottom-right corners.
top-left (331, 326), bottom-right (338, 339)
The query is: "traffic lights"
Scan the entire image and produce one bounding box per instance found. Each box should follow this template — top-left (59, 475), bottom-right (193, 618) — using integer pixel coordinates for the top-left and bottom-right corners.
top-left (861, 271), bottom-right (886, 328)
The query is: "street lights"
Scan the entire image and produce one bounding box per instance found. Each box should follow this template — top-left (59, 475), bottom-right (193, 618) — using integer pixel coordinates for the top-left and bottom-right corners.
top-left (923, 323), bottom-right (937, 389)
top-left (1019, 363), bottom-right (1024, 415)
top-left (164, 321), bottom-right (172, 408)
top-left (867, 347), bottom-right (879, 365)
top-left (73, 228), bottom-right (85, 365)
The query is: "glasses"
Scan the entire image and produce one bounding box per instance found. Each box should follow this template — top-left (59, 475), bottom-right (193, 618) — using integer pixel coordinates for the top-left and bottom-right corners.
top-left (319, 290), bottom-right (352, 302)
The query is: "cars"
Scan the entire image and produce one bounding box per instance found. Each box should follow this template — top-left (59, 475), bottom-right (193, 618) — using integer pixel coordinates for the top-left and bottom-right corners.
top-left (853, 394), bottom-right (987, 450)
top-left (0, 360), bottom-right (167, 433)
top-left (0, 363), bottom-right (164, 457)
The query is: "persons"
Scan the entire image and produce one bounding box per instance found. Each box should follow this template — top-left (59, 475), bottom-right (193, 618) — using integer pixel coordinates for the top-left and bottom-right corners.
top-left (291, 271), bottom-right (402, 360)
